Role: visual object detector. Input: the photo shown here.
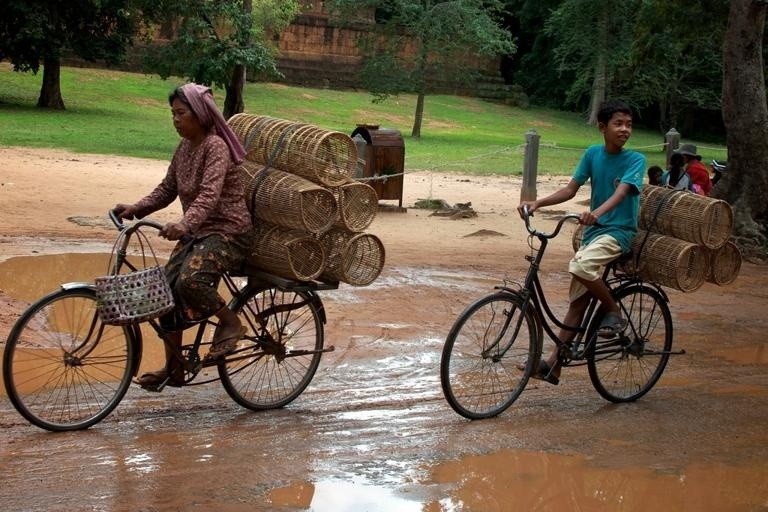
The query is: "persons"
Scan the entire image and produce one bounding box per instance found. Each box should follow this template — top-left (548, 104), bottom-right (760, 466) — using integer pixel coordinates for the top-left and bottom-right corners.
top-left (517, 103), bottom-right (645, 385)
top-left (116, 82), bottom-right (253, 391)
top-left (645, 165), bottom-right (666, 187)
top-left (662, 153), bottom-right (691, 191)
top-left (710, 159), bottom-right (728, 186)
top-left (683, 144), bottom-right (715, 193)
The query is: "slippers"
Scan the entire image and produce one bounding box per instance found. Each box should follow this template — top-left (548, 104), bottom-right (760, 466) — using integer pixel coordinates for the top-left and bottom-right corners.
top-left (516, 359), bottom-right (559, 385)
top-left (206, 325), bottom-right (248, 357)
top-left (132, 370), bottom-right (185, 388)
top-left (596, 315), bottom-right (627, 336)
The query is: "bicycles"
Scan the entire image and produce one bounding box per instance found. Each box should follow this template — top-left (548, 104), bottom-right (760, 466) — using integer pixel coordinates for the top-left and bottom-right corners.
top-left (440, 205), bottom-right (685, 420)
top-left (3, 206), bottom-right (335, 431)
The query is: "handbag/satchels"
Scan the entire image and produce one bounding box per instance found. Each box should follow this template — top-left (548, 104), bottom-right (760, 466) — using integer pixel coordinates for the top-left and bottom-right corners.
top-left (93, 225), bottom-right (176, 327)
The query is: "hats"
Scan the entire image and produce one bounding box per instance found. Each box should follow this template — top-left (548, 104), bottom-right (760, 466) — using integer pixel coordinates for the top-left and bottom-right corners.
top-left (672, 143), bottom-right (702, 162)
